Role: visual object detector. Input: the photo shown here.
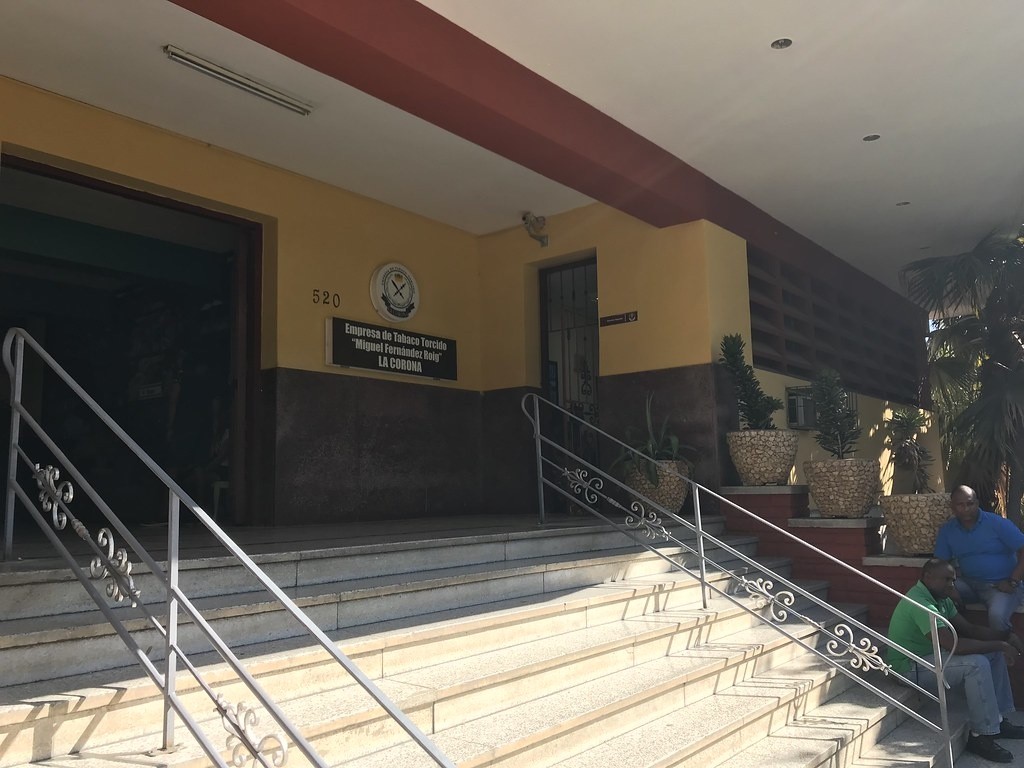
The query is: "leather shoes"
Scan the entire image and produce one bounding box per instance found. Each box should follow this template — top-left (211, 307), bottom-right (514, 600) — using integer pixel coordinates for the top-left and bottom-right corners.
top-left (993, 716), bottom-right (1024, 740)
top-left (965, 729), bottom-right (1013, 761)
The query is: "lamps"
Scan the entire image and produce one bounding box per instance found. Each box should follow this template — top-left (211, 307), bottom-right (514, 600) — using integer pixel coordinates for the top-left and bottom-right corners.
top-left (163, 44), bottom-right (315, 116)
top-left (522, 213), bottom-right (549, 247)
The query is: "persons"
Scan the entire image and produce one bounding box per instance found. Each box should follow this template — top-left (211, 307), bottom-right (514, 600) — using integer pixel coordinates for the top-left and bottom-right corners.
top-left (141, 349), bottom-right (232, 527)
top-left (886, 557), bottom-right (1024, 763)
top-left (934, 485), bottom-right (1024, 632)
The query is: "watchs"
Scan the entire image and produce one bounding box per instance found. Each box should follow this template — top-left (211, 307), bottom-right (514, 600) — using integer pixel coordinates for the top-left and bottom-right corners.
top-left (1008, 577), bottom-right (1020, 588)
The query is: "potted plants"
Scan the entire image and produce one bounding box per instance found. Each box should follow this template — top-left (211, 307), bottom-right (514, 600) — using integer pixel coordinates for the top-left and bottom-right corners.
top-left (878, 406), bottom-right (955, 557)
top-left (717, 333), bottom-right (799, 486)
top-left (801, 361), bottom-right (880, 519)
top-left (605, 392), bottom-right (706, 517)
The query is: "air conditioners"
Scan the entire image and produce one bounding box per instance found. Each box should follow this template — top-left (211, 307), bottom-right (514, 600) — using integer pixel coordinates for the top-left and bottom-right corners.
top-left (789, 395), bottom-right (817, 427)
